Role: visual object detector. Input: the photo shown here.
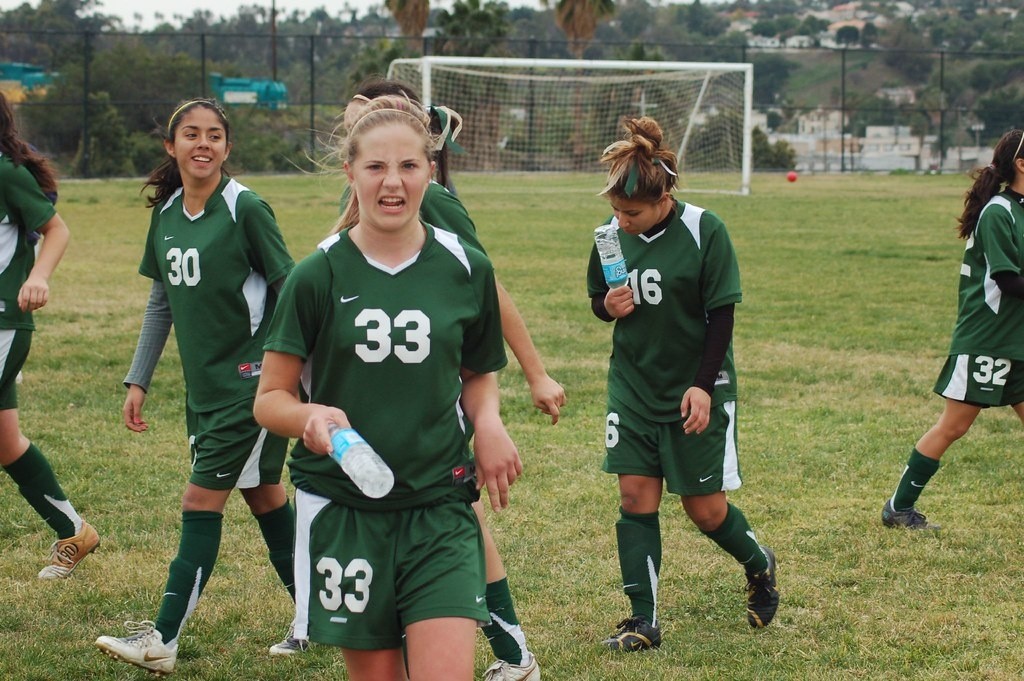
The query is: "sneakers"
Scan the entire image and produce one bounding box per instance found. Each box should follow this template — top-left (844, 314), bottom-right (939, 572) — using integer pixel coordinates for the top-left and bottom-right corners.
top-left (95, 620), bottom-right (178, 676)
top-left (601, 617), bottom-right (661, 654)
top-left (37, 520), bottom-right (100, 579)
top-left (882, 499), bottom-right (942, 531)
top-left (482, 651), bottom-right (540, 681)
top-left (745, 545), bottom-right (780, 629)
top-left (269, 618), bottom-right (309, 656)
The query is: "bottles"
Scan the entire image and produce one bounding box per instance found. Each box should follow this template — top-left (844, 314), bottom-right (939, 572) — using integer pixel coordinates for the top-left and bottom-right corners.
top-left (592, 225), bottom-right (629, 290)
top-left (325, 420), bottom-right (395, 499)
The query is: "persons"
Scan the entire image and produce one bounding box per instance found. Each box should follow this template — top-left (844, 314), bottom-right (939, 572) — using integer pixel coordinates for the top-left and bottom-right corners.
top-left (586, 116), bottom-right (779, 650)
top-left (0, 91), bottom-right (101, 579)
top-left (251, 83), bottom-right (567, 681)
top-left (881, 129), bottom-right (1024, 530)
top-left (96, 97), bottom-right (311, 673)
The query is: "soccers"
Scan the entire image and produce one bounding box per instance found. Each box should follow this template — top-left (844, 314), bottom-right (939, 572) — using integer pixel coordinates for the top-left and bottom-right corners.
top-left (787, 170), bottom-right (797, 183)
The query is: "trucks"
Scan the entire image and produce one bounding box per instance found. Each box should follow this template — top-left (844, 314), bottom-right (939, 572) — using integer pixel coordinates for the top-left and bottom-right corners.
top-left (0, 60), bottom-right (59, 93)
top-left (208, 71), bottom-right (288, 111)
